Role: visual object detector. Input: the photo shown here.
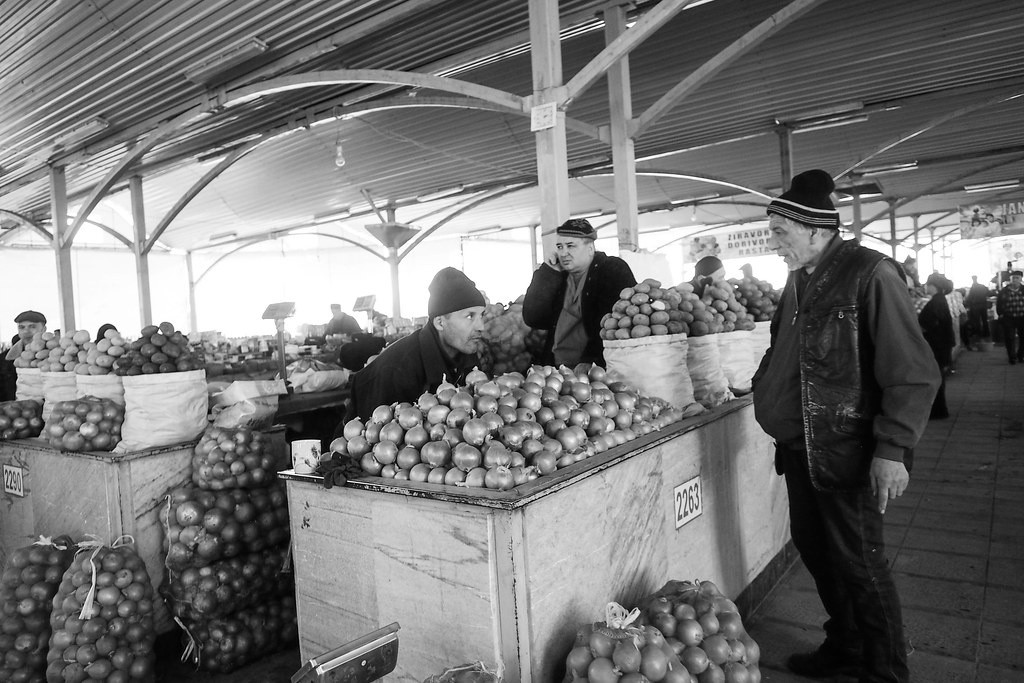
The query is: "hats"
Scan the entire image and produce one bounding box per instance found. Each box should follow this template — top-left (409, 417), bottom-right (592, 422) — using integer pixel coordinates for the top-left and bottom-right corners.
top-left (1009, 271), bottom-right (1023, 277)
top-left (767, 169), bottom-right (840, 228)
top-left (14, 310), bottom-right (46, 324)
top-left (557, 219), bottom-right (597, 240)
top-left (695, 256), bottom-right (723, 277)
top-left (331, 304), bottom-right (341, 309)
top-left (428, 267), bottom-right (486, 318)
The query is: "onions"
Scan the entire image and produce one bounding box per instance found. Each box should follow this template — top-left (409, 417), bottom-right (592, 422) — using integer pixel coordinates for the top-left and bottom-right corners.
top-left (562, 580), bottom-right (762, 683)
top-left (320, 362), bottom-right (678, 491)
top-left (0, 399), bottom-right (44, 440)
top-left (0, 534), bottom-right (159, 683)
top-left (161, 427), bottom-right (297, 674)
top-left (45, 397), bottom-right (125, 453)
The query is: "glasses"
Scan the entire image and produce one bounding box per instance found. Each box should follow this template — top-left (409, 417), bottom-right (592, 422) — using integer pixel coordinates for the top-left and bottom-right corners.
top-left (927, 282), bottom-right (933, 286)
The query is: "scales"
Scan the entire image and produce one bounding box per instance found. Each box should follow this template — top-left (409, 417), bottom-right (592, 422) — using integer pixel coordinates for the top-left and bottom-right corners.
top-left (353, 294), bottom-right (376, 335)
top-left (290, 620), bottom-right (402, 683)
top-left (262, 301), bottom-right (297, 396)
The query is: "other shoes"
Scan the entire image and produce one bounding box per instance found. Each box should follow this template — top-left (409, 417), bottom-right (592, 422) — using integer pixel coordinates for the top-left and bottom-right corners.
top-left (788, 648), bottom-right (863, 678)
top-left (1017, 355), bottom-right (1024, 361)
top-left (929, 409), bottom-right (944, 418)
top-left (1009, 357), bottom-right (1016, 364)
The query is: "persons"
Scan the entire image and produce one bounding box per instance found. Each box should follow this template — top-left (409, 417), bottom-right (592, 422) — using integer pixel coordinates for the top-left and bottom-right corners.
top-left (918, 274), bottom-right (955, 419)
top-left (342, 267), bottom-right (494, 430)
top-left (323, 304), bottom-right (363, 344)
top-left (95, 324), bottom-right (118, 344)
top-left (0, 309), bottom-right (60, 400)
top-left (685, 256), bottom-right (724, 299)
top-left (522, 218), bottom-right (639, 378)
top-left (944, 288), bottom-right (967, 348)
top-left (899, 256), bottom-right (921, 286)
top-left (996, 271), bottom-right (1024, 364)
top-left (750, 168), bottom-right (944, 683)
top-left (964, 276), bottom-right (993, 341)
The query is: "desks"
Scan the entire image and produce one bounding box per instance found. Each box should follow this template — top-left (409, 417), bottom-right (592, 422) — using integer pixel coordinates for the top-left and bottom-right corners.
top-left (0, 422), bottom-right (290, 639)
top-left (273, 389), bottom-right (801, 683)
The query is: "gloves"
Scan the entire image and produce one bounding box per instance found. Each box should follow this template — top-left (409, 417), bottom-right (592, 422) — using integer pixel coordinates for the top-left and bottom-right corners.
top-left (315, 451), bottom-right (370, 489)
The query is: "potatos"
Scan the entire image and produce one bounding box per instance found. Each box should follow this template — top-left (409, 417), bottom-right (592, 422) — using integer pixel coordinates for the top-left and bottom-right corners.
top-left (600, 276), bottom-right (781, 340)
top-left (476, 291), bottom-right (547, 373)
top-left (914, 290), bottom-right (967, 318)
top-left (13, 323), bottom-right (202, 377)
